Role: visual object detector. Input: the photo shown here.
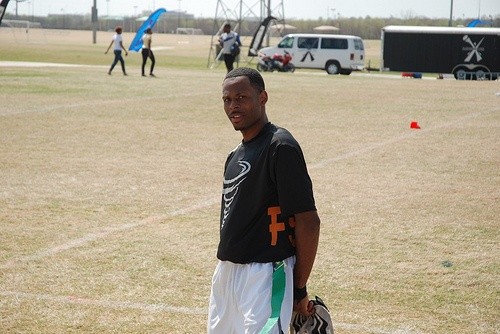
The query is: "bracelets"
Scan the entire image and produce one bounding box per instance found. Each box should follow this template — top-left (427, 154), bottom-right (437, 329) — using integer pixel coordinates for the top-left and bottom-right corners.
top-left (293, 285), bottom-right (307, 302)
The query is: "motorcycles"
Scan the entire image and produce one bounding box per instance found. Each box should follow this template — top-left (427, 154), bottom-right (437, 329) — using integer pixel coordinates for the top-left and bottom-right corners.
top-left (254, 49), bottom-right (298, 74)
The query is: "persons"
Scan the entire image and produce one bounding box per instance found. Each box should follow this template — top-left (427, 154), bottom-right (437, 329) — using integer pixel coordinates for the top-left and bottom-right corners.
top-left (141, 28), bottom-right (156, 76)
top-left (104, 26), bottom-right (128, 75)
top-left (218, 23), bottom-right (240, 73)
top-left (207, 69), bottom-right (320, 334)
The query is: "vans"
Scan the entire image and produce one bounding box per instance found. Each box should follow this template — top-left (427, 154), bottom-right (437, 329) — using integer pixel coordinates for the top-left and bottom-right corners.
top-left (259, 33), bottom-right (368, 75)
